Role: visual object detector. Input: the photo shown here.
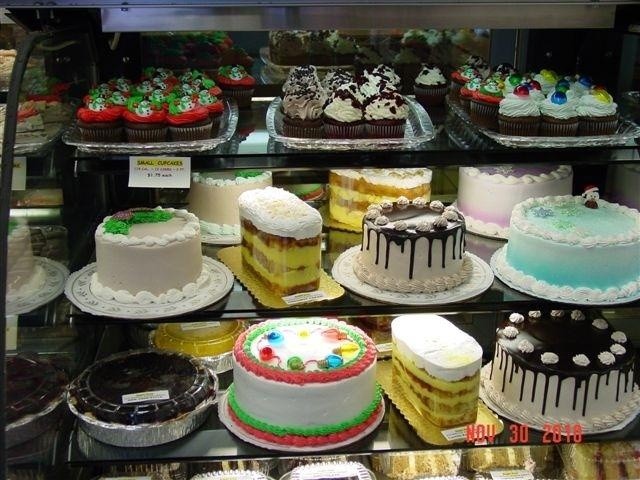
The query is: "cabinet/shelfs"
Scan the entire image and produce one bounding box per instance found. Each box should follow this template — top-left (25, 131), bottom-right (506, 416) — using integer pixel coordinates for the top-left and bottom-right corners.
top-left (0, 1), bottom-right (639, 480)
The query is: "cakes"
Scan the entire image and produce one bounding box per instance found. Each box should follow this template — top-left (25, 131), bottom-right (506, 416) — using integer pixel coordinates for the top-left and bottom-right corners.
top-left (279, 456), bottom-right (372, 480)
top-left (386, 313), bottom-right (485, 426)
top-left (129, 30), bottom-right (256, 112)
top-left (99, 454), bottom-right (183, 479)
top-left (455, 164), bottom-right (573, 241)
top-left (381, 24), bottom-right (489, 83)
top-left (325, 169), bottom-right (430, 229)
top-left (1, 348), bottom-right (64, 444)
top-left (496, 189), bottom-right (640, 301)
top-left (87, 204), bottom-right (211, 304)
top-left (266, 27), bottom-right (369, 87)
top-left (229, 316), bottom-right (384, 446)
top-left (0, 218), bottom-right (46, 303)
top-left (65, 344), bottom-right (219, 447)
top-left (414, 64), bottom-right (453, 109)
top-left (553, 436), bottom-right (640, 479)
top-left (193, 458), bottom-right (281, 478)
top-left (182, 168), bottom-right (276, 244)
top-left (77, 56), bottom-right (255, 140)
top-left (237, 185), bottom-right (325, 293)
top-left (357, 195), bottom-right (474, 295)
top-left (452, 48), bottom-right (623, 137)
top-left (0, 50), bottom-right (65, 149)
top-left (467, 441), bottom-right (555, 479)
top-left (280, 64), bottom-right (407, 137)
top-left (370, 448), bottom-right (461, 478)
top-left (480, 305), bottom-right (639, 435)
